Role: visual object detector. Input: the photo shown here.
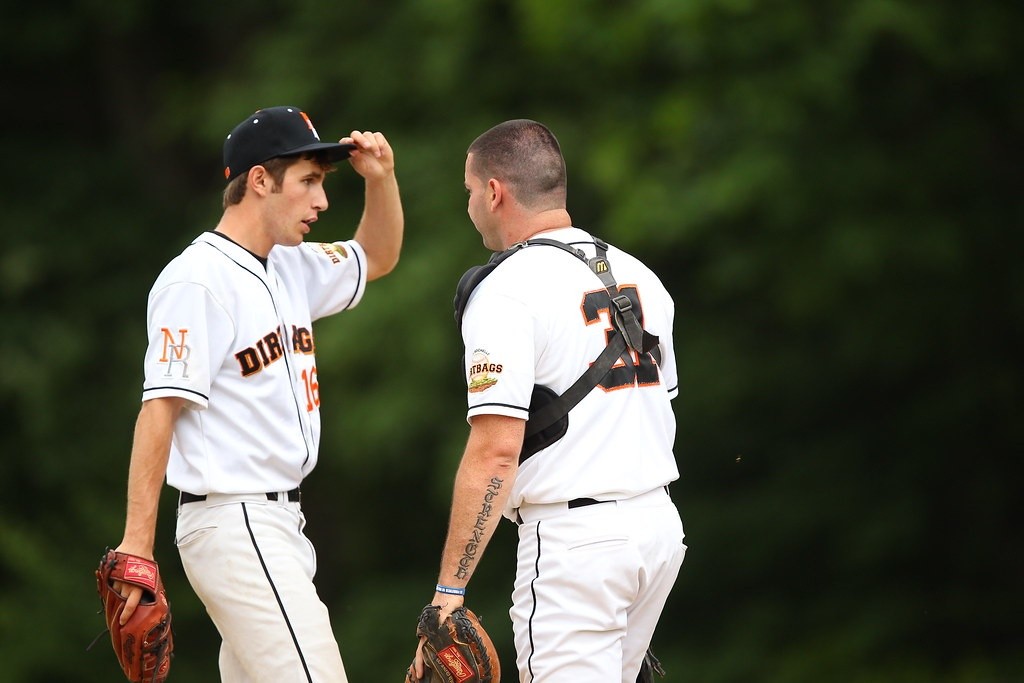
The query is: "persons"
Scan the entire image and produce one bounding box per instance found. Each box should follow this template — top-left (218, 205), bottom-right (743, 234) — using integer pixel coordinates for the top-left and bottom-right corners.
top-left (114, 105), bottom-right (402, 683)
top-left (402, 120), bottom-right (686, 683)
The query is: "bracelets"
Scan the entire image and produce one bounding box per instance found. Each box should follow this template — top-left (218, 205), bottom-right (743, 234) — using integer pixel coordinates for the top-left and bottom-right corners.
top-left (435, 584), bottom-right (466, 597)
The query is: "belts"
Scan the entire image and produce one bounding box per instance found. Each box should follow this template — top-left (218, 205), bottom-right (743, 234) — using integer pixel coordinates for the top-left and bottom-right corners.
top-left (179, 488), bottom-right (300, 506)
top-left (515, 496), bottom-right (616, 525)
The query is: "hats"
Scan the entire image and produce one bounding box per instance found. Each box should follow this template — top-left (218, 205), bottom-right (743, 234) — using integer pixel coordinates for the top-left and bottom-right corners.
top-left (223, 106), bottom-right (357, 187)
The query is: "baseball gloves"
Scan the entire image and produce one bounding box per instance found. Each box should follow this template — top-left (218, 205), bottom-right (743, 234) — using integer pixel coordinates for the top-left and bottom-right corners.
top-left (95, 546), bottom-right (174, 683)
top-left (404, 603), bottom-right (501, 683)
